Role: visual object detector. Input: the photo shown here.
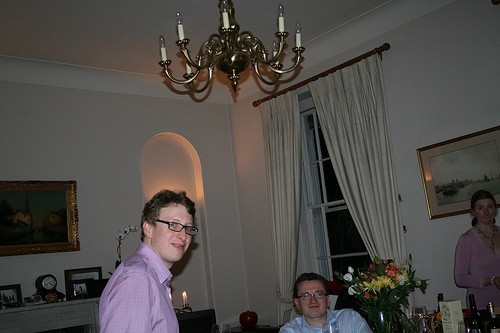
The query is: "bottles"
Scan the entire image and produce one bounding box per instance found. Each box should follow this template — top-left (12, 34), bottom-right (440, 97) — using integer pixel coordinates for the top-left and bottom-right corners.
top-left (463, 294), bottom-right (500, 333)
top-left (435, 293), bottom-right (446, 333)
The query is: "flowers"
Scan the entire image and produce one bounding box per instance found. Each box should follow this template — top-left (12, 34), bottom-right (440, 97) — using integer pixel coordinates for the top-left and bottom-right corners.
top-left (333, 254), bottom-right (431, 333)
top-left (106, 225), bottom-right (139, 281)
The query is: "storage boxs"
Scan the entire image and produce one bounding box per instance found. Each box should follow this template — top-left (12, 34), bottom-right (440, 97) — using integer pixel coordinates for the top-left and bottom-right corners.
top-left (439, 299), bottom-right (465, 333)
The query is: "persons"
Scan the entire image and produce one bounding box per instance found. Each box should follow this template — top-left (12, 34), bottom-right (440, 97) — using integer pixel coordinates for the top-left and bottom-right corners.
top-left (455, 191), bottom-right (500, 310)
top-left (98, 190), bottom-right (199, 333)
top-left (279, 273), bottom-right (372, 333)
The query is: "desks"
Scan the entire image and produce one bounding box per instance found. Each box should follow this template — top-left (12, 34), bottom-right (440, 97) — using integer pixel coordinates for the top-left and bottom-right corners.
top-left (176, 309), bottom-right (217, 333)
top-left (226, 325), bottom-right (270, 333)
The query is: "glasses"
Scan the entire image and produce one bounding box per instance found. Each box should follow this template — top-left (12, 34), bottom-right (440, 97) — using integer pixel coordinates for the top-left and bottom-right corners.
top-left (297, 290), bottom-right (327, 300)
top-left (153, 219), bottom-right (199, 236)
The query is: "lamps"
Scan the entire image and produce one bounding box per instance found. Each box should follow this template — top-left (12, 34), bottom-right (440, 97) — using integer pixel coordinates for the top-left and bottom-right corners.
top-left (158, 0), bottom-right (305, 93)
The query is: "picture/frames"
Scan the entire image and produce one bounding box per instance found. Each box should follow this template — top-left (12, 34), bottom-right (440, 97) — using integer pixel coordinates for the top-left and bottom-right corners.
top-left (417, 125), bottom-right (500, 220)
top-left (0, 181), bottom-right (80, 257)
top-left (0, 284), bottom-right (23, 310)
top-left (65, 267), bottom-right (102, 301)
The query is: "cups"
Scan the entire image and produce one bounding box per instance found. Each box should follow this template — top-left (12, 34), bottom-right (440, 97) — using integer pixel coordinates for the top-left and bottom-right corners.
top-left (322, 320), bottom-right (340, 333)
top-left (413, 306), bottom-right (428, 330)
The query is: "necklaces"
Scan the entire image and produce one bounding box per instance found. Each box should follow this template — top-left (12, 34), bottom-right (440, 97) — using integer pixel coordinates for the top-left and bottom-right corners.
top-left (476, 225), bottom-right (496, 247)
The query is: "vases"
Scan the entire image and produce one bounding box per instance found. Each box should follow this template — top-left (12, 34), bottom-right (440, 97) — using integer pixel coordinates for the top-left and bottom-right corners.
top-left (374, 311), bottom-right (392, 333)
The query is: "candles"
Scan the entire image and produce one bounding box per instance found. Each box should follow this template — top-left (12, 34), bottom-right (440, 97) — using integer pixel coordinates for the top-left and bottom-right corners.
top-left (182, 291), bottom-right (188, 307)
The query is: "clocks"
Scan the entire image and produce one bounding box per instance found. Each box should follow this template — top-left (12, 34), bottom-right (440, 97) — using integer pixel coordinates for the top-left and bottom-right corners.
top-left (33, 274), bottom-right (65, 304)
